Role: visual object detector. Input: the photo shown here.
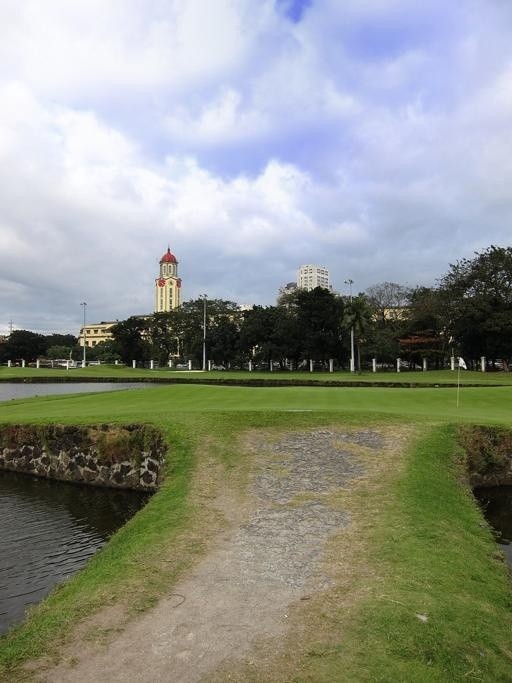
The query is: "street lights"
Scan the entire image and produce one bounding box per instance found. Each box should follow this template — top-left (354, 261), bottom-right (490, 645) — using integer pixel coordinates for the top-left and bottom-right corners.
top-left (343, 278), bottom-right (355, 372)
top-left (80, 302), bottom-right (88, 367)
top-left (199, 293), bottom-right (209, 370)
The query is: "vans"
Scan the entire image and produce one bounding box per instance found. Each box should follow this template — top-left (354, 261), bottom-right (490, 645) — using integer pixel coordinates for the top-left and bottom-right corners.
top-left (442, 356), bottom-right (469, 370)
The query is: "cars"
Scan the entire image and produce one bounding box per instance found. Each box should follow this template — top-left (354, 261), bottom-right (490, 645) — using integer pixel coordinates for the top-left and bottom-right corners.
top-left (368, 360), bottom-right (424, 371)
top-left (139, 361), bottom-right (161, 370)
top-left (11, 358), bottom-right (105, 371)
top-left (485, 358), bottom-right (512, 372)
top-left (176, 362), bottom-right (189, 369)
top-left (213, 358), bottom-right (334, 372)
